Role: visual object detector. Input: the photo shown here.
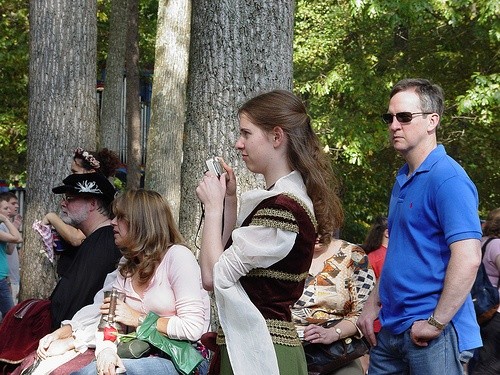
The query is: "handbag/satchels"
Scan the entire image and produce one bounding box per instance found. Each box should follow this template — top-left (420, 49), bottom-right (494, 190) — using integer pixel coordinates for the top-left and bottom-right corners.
top-left (117, 338), bottom-right (153, 358)
top-left (301, 319), bottom-right (371, 374)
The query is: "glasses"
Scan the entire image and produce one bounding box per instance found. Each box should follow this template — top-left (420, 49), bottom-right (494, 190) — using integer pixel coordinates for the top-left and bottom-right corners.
top-left (382, 111), bottom-right (433, 124)
top-left (61, 195), bottom-right (77, 202)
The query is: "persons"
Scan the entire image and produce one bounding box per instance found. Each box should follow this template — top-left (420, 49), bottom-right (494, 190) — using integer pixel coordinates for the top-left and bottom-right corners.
top-left (356, 78), bottom-right (483, 375)
top-left (0, 147), bottom-right (214, 375)
top-left (195, 89), bottom-right (339, 375)
top-left (287, 210), bottom-right (500, 375)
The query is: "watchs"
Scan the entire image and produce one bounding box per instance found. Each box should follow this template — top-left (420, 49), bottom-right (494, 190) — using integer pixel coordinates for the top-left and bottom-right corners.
top-left (333, 326), bottom-right (341, 339)
top-left (428, 313), bottom-right (447, 330)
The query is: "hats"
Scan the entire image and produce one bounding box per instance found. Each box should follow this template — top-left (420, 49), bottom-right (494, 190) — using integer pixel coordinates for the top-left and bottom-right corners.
top-left (51, 173), bottom-right (115, 204)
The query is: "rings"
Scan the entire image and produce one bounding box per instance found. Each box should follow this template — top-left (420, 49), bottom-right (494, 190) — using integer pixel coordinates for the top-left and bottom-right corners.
top-left (98, 369), bottom-right (103, 372)
top-left (109, 362), bottom-right (116, 366)
top-left (318, 333), bottom-right (321, 338)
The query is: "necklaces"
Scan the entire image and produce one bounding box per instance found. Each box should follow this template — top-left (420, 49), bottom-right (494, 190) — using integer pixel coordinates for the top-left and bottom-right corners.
top-left (86, 216), bottom-right (109, 235)
top-left (265, 182), bottom-right (275, 192)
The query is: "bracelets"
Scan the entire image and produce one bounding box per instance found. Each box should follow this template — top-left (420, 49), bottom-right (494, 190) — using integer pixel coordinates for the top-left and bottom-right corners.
top-left (138, 313), bottom-right (146, 325)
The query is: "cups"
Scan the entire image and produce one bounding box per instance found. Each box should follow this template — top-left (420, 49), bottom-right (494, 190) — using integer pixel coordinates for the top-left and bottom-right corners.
top-left (52, 225), bottom-right (66, 251)
top-left (98, 289), bottom-right (127, 334)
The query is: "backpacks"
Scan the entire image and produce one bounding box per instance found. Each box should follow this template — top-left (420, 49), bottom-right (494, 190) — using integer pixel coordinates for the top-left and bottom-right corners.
top-left (470, 237), bottom-right (500, 317)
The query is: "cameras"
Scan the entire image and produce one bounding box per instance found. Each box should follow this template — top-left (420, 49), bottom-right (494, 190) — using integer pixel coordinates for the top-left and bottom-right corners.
top-left (205, 159), bottom-right (224, 178)
